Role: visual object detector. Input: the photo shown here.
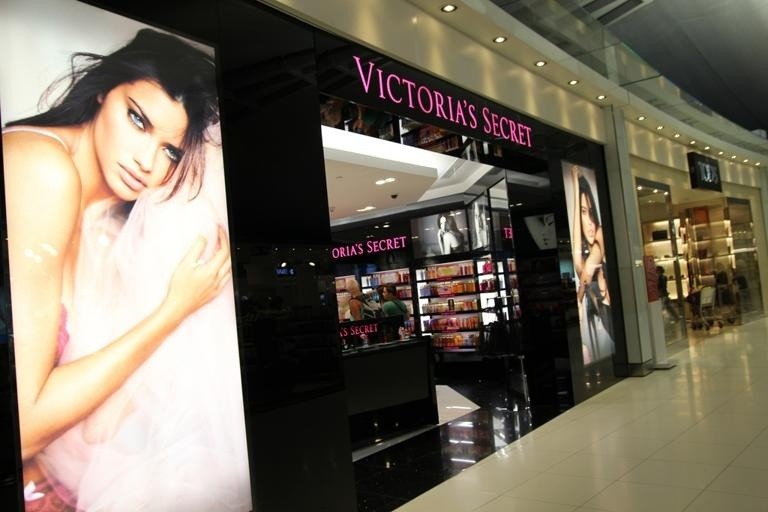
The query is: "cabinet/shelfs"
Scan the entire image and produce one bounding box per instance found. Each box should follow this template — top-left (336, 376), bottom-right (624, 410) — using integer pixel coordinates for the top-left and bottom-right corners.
top-left (333, 258), bottom-right (522, 355)
top-left (639, 198), bottom-right (759, 301)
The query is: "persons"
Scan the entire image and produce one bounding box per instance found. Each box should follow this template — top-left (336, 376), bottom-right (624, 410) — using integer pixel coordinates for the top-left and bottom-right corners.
top-left (1, 26), bottom-right (234, 512)
top-left (540, 213), bottom-right (558, 249)
top-left (376, 284), bottom-right (407, 328)
top-left (567, 165), bottom-right (622, 365)
top-left (468, 202), bottom-right (489, 247)
top-left (431, 214), bottom-right (468, 256)
top-left (345, 280), bottom-right (381, 320)
top-left (656, 257), bottom-right (680, 327)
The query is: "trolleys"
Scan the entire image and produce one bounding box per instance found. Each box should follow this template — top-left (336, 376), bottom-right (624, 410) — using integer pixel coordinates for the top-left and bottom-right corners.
top-left (684, 287), bottom-right (725, 333)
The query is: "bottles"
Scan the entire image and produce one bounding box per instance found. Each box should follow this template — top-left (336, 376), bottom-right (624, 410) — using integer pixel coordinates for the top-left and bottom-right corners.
top-left (420, 259), bottom-right (492, 347)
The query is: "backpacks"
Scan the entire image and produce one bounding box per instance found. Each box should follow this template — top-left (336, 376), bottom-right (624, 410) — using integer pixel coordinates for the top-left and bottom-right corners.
top-left (349, 294), bottom-right (385, 319)
